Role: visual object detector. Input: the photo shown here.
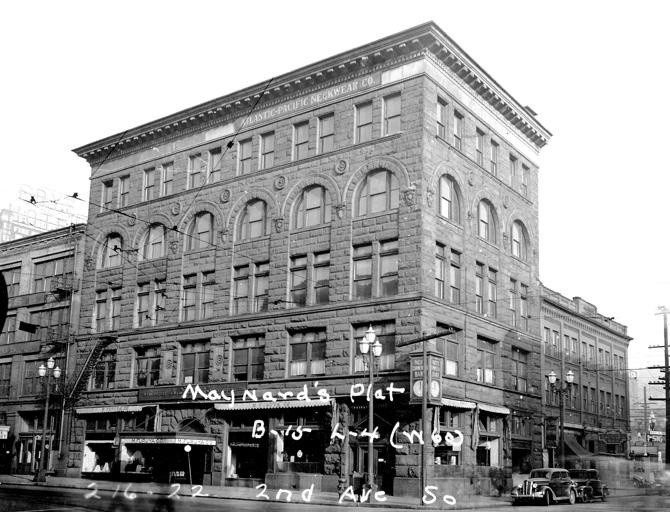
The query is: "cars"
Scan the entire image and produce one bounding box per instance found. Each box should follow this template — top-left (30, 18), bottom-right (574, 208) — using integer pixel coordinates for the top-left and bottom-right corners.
top-left (511, 467), bottom-right (579, 506)
top-left (568, 469), bottom-right (610, 503)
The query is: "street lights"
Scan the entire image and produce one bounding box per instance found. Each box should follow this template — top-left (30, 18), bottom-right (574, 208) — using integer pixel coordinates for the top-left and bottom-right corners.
top-left (32, 355), bottom-right (61, 483)
top-left (549, 369), bottom-right (575, 469)
top-left (359, 323), bottom-right (382, 504)
